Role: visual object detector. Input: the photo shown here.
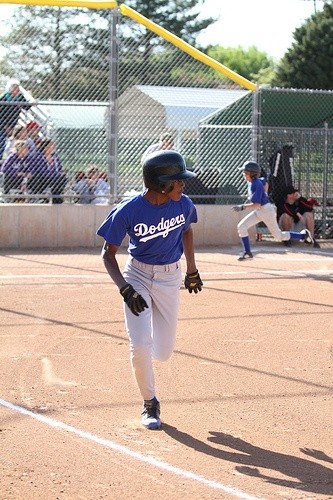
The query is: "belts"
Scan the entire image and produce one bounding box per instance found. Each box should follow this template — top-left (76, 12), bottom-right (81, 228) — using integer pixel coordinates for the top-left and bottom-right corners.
top-left (128, 255), bottom-right (180, 272)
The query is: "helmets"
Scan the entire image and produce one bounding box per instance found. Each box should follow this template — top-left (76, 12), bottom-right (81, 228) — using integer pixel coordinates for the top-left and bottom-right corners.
top-left (238, 161), bottom-right (260, 172)
top-left (143, 151), bottom-right (196, 186)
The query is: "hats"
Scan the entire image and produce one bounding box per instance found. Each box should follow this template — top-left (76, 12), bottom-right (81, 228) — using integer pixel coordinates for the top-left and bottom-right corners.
top-left (27, 120), bottom-right (42, 129)
top-left (40, 138), bottom-right (52, 150)
top-left (283, 186), bottom-right (298, 194)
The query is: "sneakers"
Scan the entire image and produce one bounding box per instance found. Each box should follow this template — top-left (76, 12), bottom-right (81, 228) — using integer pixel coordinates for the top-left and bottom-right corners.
top-left (301, 230), bottom-right (321, 248)
top-left (237, 252), bottom-right (253, 261)
top-left (141, 397), bottom-right (161, 430)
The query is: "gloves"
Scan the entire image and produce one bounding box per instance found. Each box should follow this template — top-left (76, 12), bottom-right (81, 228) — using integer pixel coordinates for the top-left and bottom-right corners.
top-left (231, 203), bottom-right (246, 212)
top-left (185, 270), bottom-right (203, 294)
top-left (120, 284), bottom-right (149, 317)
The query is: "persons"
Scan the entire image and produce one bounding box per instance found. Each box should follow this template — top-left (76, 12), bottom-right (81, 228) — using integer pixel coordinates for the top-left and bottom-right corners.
top-left (95, 150), bottom-right (203, 430)
top-left (279, 187), bottom-right (321, 249)
top-left (0, 121), bottom-right (69, 204)
top-left (0, 77), bottom-right (37, 160)
top-left (71, 167), bottom-right (109, 204)
top-left (141, 133), bottom-right (179, 192)
top-left (231, 161), bottom-right (314, 261)
